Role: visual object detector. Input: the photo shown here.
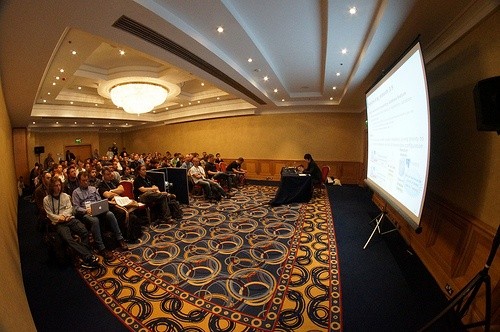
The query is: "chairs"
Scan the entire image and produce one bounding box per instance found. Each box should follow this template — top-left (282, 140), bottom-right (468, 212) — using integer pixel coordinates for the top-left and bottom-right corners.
top-left (37, 160), bottom-right (245, 262)
top-left (312, 166), bottom-right (329, 194)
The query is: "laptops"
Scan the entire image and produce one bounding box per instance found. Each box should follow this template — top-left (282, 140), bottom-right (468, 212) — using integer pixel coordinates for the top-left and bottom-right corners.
top-left (87, 199), bottom-right (109, 216)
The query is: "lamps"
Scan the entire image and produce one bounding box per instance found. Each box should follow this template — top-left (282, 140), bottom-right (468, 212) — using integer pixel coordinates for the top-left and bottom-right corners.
top-left (97, 77), bottom-right (181, 116)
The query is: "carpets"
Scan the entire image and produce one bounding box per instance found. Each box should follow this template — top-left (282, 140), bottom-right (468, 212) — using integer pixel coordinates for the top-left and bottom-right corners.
top-left (67, 181), bottom-right (342, 332)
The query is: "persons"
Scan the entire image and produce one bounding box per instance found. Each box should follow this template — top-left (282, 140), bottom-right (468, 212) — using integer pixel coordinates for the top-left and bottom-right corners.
top-left (301, 151), bottom-right (324, 186)
top-left (27, 141), bottom-right (247, 269)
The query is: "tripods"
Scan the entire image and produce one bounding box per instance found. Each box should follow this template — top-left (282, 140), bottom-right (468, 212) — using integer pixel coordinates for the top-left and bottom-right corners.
top-left (418, 224), bottom-right (500, 332)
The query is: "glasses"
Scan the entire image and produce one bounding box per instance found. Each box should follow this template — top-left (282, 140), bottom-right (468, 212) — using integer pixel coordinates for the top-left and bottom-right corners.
top-left (46, 176), bottom-right (52, 179)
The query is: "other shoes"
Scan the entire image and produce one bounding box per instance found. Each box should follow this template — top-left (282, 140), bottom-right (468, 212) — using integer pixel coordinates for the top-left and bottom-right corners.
top-left (119, 239), bottom-right (129, 251)
top-left (165, 218), bottom-right (176, 223)
top-left (81, 263), bottom-right (96, 268)
top-left (90, 254), bottom-right (100, 263)
top-left (102, 250), bottom-right (112, 259)
top-left (227, 192), bottom-right (236, 198)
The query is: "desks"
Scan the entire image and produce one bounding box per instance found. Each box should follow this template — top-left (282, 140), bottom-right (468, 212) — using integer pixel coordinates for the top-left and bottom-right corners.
top-left (279, 169), bottom-right (311, 201)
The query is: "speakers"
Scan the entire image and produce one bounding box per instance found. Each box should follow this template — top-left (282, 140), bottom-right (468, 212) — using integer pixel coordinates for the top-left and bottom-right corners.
top-left (474, 76), bottom-right (500, 132)
top-left (34, 146), bottom-right (44, 154)
